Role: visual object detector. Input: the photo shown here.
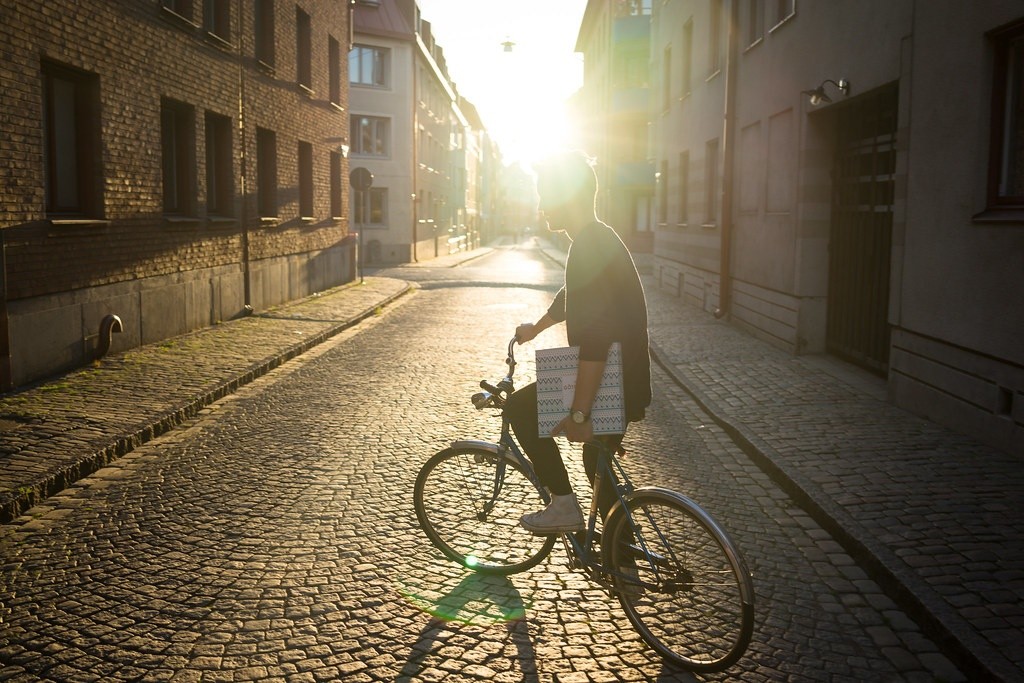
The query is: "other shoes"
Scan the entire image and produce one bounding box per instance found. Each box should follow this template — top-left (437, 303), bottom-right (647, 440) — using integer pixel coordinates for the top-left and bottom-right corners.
top-left (520, 493), bottom-right (585, 532)
top-left (615, 568), bottom-right (645, 604)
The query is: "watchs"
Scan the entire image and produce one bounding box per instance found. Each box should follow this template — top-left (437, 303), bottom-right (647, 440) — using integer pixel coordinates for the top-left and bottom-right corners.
top-left (569, 406), bottom-right (592, 424)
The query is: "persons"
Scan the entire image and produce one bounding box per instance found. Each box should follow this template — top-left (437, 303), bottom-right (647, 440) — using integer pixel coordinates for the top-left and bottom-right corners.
top-left (505, 159), bottom-right (652, 601)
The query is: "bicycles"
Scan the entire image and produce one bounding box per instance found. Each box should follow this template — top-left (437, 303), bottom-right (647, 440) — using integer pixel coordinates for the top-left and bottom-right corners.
top-left (412, 335), bottom-right (758, 673)
